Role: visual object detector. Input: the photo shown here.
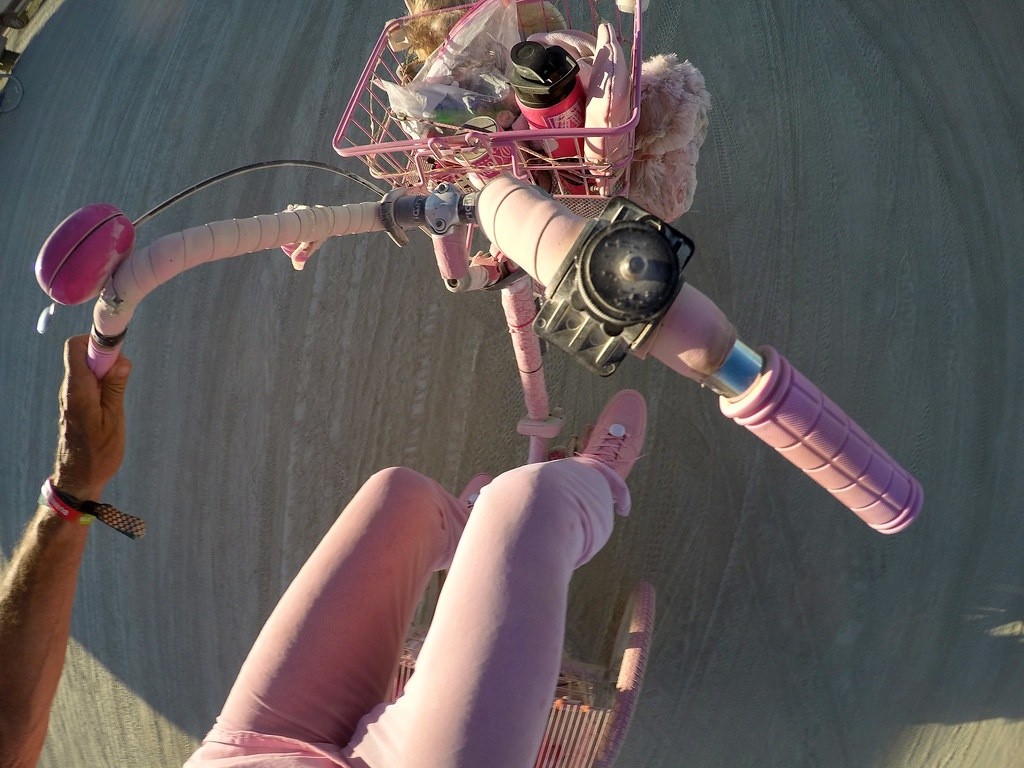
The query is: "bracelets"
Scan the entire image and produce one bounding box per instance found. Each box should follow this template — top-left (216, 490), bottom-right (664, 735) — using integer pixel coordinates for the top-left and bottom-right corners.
top-left (37, 476), bottom-right (146, 541)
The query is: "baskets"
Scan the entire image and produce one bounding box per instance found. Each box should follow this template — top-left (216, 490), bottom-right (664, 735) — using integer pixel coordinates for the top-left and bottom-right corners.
top-left (331, 0), bottom-right (643, 218)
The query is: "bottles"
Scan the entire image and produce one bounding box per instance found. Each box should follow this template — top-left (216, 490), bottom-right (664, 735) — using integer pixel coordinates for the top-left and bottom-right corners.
top-left (507, 41), bottom-right (600, 195)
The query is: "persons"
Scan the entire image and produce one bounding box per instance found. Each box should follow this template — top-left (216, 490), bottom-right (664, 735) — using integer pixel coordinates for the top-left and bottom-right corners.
top-left (0, 334), bottom-right (647, 768)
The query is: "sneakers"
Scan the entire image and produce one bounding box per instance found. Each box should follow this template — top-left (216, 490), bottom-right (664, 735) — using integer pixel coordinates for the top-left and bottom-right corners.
top-left (458, 473), bottom-right (494, 511)
top-left (575, 388), bottom-right (649, 482)
